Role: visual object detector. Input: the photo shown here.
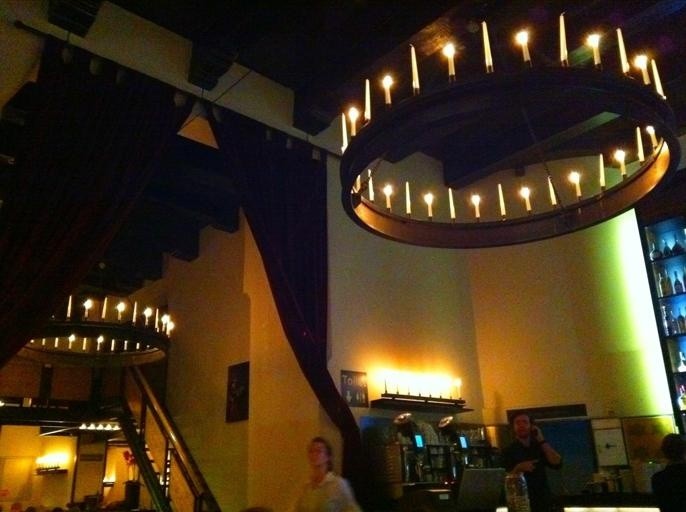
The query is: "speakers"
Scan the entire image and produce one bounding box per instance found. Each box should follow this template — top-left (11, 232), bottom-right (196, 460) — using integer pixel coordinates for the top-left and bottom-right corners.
top-left (189, 14), bottom-right (241, 91)
top-left (293, 78), bottom-right (340, 136)
top-left (47, 0), bottom-right (107, 38)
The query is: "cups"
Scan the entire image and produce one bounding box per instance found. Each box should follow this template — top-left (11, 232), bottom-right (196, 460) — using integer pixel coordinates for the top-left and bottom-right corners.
top-left (505, 472), bottom-right (530, 512)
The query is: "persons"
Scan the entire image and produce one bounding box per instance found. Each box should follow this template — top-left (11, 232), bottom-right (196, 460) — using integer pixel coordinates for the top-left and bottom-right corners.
top-left (650, 432), bottom-right (686, 512)
top-left (288, 435), bottom-right (363, 511)
top-left (502, 410), bottom-right (562, 512)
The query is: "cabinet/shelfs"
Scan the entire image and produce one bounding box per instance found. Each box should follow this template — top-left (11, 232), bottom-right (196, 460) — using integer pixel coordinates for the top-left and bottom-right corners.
top-left (634, 170), bottom-right (686, 435)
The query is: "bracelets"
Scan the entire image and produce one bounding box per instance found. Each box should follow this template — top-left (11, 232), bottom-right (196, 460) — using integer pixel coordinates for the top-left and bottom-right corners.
top-left (538, 439), bottom-right (547, 445)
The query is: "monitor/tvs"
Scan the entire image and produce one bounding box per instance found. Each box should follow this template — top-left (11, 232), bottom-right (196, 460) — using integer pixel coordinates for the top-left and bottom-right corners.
top-left (458, 435), bottom-right (469, 450)
top-left (414, 432), bottom-right (424, 449)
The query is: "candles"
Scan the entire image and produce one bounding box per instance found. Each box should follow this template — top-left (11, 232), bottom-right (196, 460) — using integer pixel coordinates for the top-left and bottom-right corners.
top-left (615, 150), bottom-right (626, 175)
top-left (616, 28), bottom-right (629, 73)
top-left (548, 177), bottom-right (557, 206)
top-left (31, 294), bottom-right (176, 353)
top-left (341, 112), bottom-right (348, 154)
top-left (349, 107), bottom-right (357, 136)
top-left (428, 196), bottom-right (433, 217)
top-left (443, 44), bottom-right (455, 76)
top-left (356, 173), bottom-right (361, 192)
top-left (449, 188), bottom-right (455, 219)
top-left (481, 21), bottom-right (493, 66)
top-left (587, 34), bottom-right (601, 65)
top-left (368, 168), bottom-right (374, 200)
top-left (651, 59), bottom-right (664, 97)
top-left (646, 125), bottom-right (658, 151)
top-left (365, 79), bottom-right (371, 120)
top-left (637, 127), bottom-right (645, 162)
top-left (405, 182), bottom-right (411, 215)
top-left (384, 184), bottom-right (392, 208)
top-left (410, 43), bottom-right (419, 88)
top-left (635, 56), bottom-right (651, 85)
top-left (520, 187), bottom-right (533, 211)
top-left (568, 172), bottom-right (583, 197)
top-left (559, 12), bottom-right (568, 62)
top-left (472, 196), bottom-right (480, 217)
top-left (498, 183), bottom-right (505, 216)
top-left (383, 76), bottom-right (392, 104)
top-left (515, 32), bottom-right (530, 63)
top-left (600, 153), bottom-right (606, 186)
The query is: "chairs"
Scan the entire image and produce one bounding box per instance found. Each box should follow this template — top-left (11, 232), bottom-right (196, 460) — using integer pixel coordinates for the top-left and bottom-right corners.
top-left (455, 468), bottom-right (509, 512)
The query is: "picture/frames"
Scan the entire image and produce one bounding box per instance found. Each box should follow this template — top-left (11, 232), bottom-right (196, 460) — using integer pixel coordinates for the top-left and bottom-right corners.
top-left (341, 368), bottom-right (370, 409)
top-left (225, 361), bottom-right (251, 423)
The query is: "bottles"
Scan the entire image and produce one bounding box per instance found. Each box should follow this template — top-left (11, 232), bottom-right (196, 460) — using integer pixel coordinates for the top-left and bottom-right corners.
top-left (650, 235), bottom-right (686, 411)
